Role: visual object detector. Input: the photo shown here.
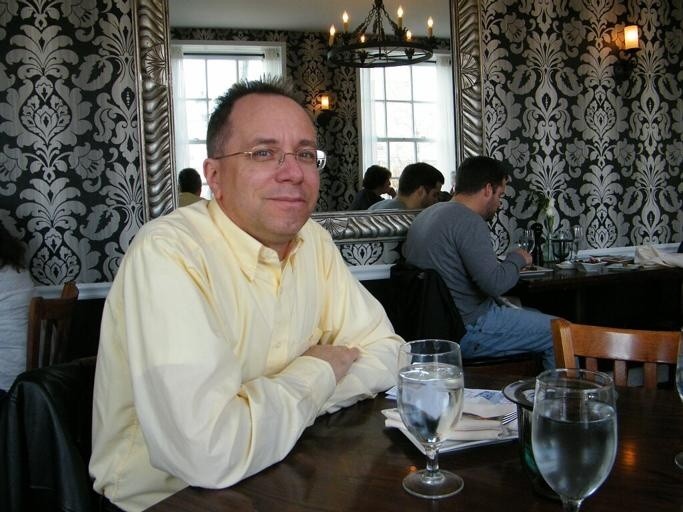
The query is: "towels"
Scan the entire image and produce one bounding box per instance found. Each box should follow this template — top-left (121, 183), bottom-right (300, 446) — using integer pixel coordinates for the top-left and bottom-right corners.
top-left (374, 400), bottom-right (519, 441)
top-left (632, 244), bottom-right (683, 270)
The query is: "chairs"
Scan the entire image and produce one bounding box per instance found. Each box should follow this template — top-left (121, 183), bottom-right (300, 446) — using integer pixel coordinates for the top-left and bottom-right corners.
top-left (547, 317), bottom-right (683, 397)
top-left (22, 279), bottom-right (81, 371)
top-left (383, 253), bottom-right (543, 379)
top-left (8, 351), bottom-right (102, 511)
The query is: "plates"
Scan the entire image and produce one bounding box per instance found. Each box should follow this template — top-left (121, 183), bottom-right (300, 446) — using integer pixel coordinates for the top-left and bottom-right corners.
top-left (496, 256), bottom-right (656, 276)
top-left (381, 397), bottom-right (522, 455)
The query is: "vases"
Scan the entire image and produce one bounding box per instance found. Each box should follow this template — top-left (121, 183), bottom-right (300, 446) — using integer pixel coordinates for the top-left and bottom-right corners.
top-left (541, 233), bottom-right (555, 267)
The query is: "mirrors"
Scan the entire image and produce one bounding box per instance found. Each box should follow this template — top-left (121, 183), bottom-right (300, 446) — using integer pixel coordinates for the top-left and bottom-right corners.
top-left (127, 1), bottom-right (491, 247)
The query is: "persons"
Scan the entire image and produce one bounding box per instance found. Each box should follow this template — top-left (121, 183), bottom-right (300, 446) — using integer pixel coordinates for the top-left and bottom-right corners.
top-left (89, 73), bottom-right (412, 512)
top-left (368, 163), bottom-right (443, 211)
top-left (402, 158), bottom-right (581, 377)
top-left (348, 165), bottom-right (397, 210)
top-left (176, 168), bottom-right (206, 207)
top-left (0, 219), bottom-right (56, 396)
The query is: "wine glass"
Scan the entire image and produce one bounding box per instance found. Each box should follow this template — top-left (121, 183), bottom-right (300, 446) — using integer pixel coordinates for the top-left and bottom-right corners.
top-left (396, 340), bottom-right (465, 500)
top-left (531, 368), bottom-right (618, 512)
top-left (675, 329), bottom-right (683, 469)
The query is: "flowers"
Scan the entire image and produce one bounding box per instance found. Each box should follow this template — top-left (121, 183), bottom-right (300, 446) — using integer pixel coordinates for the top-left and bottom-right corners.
top-left (544, 197), bottom-right (556, 267)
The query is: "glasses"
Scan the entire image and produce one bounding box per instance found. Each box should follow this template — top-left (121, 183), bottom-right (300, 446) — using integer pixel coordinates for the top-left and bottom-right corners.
top-left (213, 146), bottom-right (326, 174)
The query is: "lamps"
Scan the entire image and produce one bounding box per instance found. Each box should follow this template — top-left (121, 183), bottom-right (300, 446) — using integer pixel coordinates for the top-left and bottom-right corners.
top-left (610, 21), bottom-right (641, 80)
top-left (322, 1), bottom-right (437, 72)
top-left (310, 93), bottom-right (333, 127)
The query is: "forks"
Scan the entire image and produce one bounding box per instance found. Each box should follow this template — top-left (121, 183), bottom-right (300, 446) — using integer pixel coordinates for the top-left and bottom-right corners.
top-left (462, 409), bottom-right (517, 425)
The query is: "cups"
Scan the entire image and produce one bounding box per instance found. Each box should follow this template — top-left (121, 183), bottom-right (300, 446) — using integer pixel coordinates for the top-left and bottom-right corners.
top-left (519, 225), bottom-right (583, 264)
top-left (503, 377), bottom-right (620, 476)
